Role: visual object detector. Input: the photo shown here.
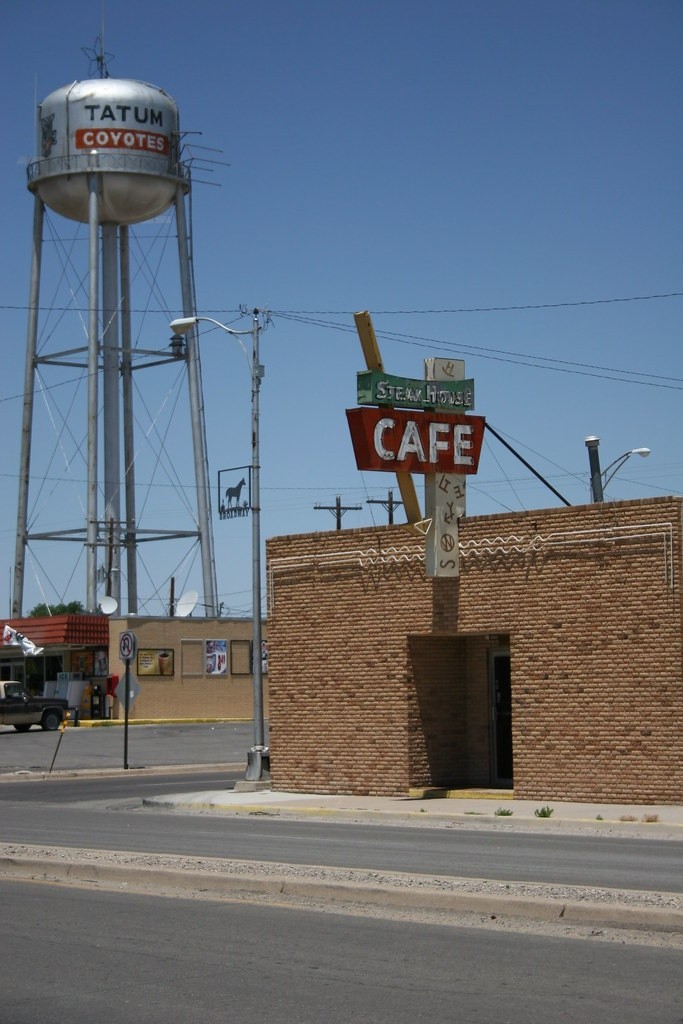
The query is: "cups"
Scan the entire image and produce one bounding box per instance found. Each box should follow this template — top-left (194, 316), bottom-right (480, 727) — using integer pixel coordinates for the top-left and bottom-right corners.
top-left (158, 653), bottom-right (169, 674)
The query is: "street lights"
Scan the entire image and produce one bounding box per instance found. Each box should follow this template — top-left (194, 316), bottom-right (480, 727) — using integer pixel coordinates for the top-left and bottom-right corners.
top-left (590, 448), bottom-right (650, 502)
top-left (170, 305), bottom-right (269, 778)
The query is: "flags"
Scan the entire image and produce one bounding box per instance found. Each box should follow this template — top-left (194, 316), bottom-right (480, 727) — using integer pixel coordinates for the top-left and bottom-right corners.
top-left (2, 625), bottom-right (44, 656)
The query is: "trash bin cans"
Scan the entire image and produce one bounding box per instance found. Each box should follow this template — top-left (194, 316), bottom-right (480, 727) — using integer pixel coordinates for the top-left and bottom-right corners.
top-left (81, 686), bottom-right (92, 720)
top-left (91, 685), bottom-right (103, 720)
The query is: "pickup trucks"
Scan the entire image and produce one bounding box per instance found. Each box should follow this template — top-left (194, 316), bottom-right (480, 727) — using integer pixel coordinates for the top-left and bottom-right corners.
top-left (0, 680), bottom-right (68, 731)
